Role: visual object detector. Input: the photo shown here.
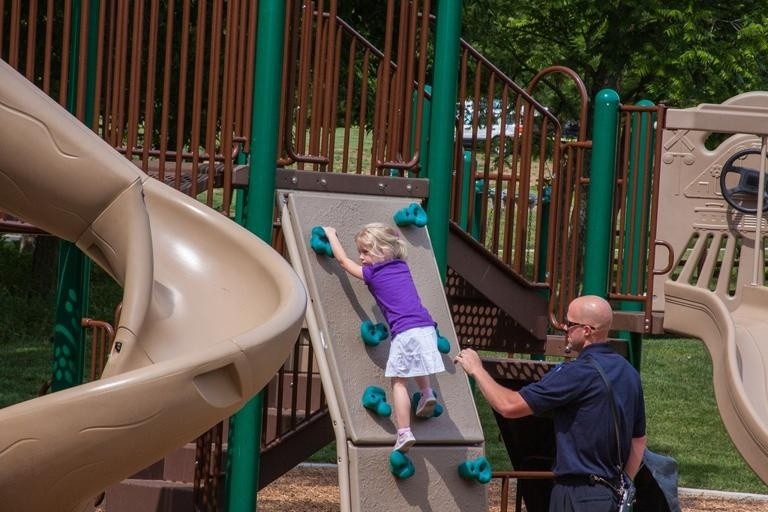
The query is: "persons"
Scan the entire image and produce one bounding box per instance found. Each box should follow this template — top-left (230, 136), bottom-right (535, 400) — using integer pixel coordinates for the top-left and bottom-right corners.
top-left (452, 294), bottom-right (646, 511)
top-left (322, 219), bottom-right (445, 452)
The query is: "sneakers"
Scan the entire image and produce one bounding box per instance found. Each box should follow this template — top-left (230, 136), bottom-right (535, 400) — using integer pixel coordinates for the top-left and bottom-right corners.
top-left (393, 428), bottom-right (415, 452)
top-left (415, 393), bottom-right (436, 418)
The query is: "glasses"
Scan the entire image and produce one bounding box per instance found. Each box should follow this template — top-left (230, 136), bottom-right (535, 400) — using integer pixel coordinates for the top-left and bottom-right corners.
top-left (567, 320), bottom-right (596, 330)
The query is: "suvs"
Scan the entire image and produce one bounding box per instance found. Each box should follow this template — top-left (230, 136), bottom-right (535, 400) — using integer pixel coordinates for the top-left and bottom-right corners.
top-left (454, 96), bottom-right (548, 158)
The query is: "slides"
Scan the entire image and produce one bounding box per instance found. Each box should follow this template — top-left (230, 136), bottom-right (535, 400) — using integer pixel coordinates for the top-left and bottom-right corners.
top-left (664, 280), bottom-right (768, 486)
top-left (0, 60), bottom-right (306, 512)
